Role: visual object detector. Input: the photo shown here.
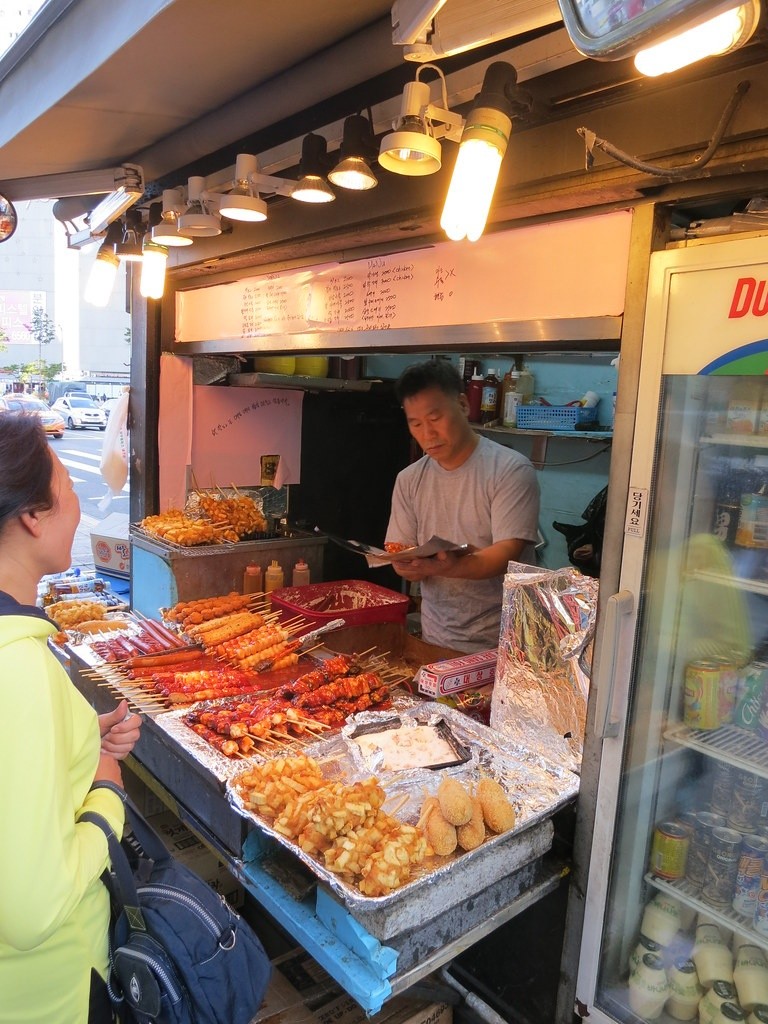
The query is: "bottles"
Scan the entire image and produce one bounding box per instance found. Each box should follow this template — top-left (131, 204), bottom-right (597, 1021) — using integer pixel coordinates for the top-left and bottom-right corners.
top-left (627, 888), bottom-right (768, 1023)
top-left (244, 559), bottom-right (262, 613)
top-left (293, 560), bottom-right (309, 586)
top-left (36, 567), bottom-right (126, 615)
top-left (265, 561), bottom-right (283, 610)
top-left (456, 354), bottom-right (535, 428)
top-left (610, 391), bottom-right (617, 430)
top-left (703, 375), bottom-right (768, 438)
top-left (254, 356), bottom-right (362, 380)
top-left (579, 391), bottom-right (601, 415)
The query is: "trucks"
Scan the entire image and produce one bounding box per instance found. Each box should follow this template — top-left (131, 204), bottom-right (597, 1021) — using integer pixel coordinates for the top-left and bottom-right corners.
top-left (48, 381), bottom-right (94, 404)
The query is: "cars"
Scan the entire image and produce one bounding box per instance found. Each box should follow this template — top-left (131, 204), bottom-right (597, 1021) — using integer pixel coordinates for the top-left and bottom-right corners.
top-left (102, 399), bottom-right (118, 418)
top-left (0, 392), bottom-right (64, 438)
top-left (51, 397), bottom-right (107, 431)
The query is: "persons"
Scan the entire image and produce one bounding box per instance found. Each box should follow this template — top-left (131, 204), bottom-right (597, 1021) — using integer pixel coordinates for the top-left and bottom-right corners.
top-left (566, 484), bottom-right (608, 579)
top-left (0, 409), bottom-right (142, 1024)
top-left (386, 360), bottom-right (541, 653)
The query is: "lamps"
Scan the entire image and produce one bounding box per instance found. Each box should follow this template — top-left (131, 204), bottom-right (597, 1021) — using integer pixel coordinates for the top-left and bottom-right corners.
top-left (152, 187), bottom-right (193, 247)
top-left (217, 153), bottom-right (278, 222)
top-left (379, 76), bottom-right (462, 178)
top-left (287, 132), bottom-right (338, 204)
top-left (178, 174), bottom-right (223, 238)
top-left (330, 105), bottom-right (380, 190)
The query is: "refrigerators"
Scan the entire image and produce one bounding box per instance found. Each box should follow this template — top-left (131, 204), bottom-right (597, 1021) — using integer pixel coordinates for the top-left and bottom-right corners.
top-left (575, 227), bottom-right (768, 1018)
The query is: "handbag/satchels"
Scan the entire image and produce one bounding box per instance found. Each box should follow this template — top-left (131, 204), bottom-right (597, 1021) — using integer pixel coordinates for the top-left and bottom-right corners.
top-left (75, 779), bottom-right (271, 1024)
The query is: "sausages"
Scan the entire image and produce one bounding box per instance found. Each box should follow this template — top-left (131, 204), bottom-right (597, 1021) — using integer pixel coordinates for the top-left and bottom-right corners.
top-left (92, 618), bottom-right (202, 679)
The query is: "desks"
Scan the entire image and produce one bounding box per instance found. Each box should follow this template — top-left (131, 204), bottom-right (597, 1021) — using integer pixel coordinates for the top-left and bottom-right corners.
top-left (42, 628), bottom-right (581, 1013)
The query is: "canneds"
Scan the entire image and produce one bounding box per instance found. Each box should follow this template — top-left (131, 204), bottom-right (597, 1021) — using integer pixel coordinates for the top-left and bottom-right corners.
top-left (650, 757), bottom-right (767, 943)
top-left (683, 649), bottom-right (768, 748)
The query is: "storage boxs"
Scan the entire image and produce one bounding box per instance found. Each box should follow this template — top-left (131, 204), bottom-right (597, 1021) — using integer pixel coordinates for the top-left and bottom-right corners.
top-left (90, 511), bottom-right (133, 581)
top-left (412, 647), bottom-right (497, 698)
top-left (133, 801), bottom-right (458, 1024)
top-left (266, 579), bottom-right (410, 631)
